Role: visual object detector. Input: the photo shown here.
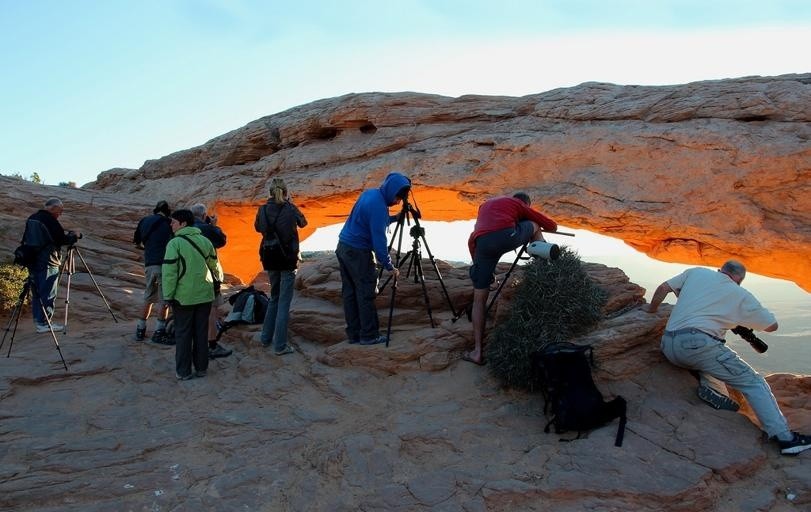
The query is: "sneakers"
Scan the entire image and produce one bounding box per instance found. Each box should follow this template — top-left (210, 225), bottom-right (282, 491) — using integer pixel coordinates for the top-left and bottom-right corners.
top-left (779, 433), bottom-right (811, 455)
top-left (207, 344), bottom-right (232, 357)
top-left (151, 329), bottom-right (163, 342)
top-left (36, 324), bottom-right (63, 333)
top-left (175, 369), bottom-right (206, 380)
top-left (697, 385), bottom-right (740, 411)
top-left (274, 345), bottom-right (293, 355)
top-left (349, 336), bottom-right (387, 345)
top-left (135, 326), bottom-right (145, 340)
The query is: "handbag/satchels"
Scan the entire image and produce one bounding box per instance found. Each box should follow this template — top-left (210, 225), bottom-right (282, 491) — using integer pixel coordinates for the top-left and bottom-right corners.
top-left (14, 244), bottom-right (34, 266)
top-left (242, 294), bottom-right (269, 325)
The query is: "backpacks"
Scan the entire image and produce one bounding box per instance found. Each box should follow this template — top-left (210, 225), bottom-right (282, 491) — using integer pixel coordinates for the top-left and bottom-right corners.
top-left (259, 203), bottom-right (289, 270)
top-left (530, 341), bottom-right (627, 447)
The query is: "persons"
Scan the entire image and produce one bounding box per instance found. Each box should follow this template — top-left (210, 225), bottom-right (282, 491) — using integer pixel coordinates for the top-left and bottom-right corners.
top-left (461, 192), bottom-right (558, 365)
top-left (134, 200), bottom-right (176, 343)
top-left (161, 208), bottom-right (224, 381)
top-left (189, 203), bottom-right (233, 358)
top-left (252, 175), bottom-right (308, 357)
top-left (21, 197), bottom-right (78, 334)
top-left (334, 171), bottom-right (413, 345)
top-left (646, 258), bottom-right (811, 456)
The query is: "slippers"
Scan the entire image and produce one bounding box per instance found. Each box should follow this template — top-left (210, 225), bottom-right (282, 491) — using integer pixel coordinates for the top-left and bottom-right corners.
top-left (462, 351), bottom-right (487, 366)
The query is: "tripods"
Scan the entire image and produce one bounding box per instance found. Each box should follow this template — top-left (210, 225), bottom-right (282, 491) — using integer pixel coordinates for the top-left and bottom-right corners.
top-left (57, 245), bottom-right (118, 335)
top-left (379, 200), bottom-right (458, 348)
top-left (481, 239), bottom-right (535, 316)
top-left (0, 278), bottom-right (68, 371)
top-left (377, 237), bottom-right (438, 329)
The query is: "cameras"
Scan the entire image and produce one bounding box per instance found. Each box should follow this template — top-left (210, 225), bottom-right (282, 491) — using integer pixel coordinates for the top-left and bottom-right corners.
top-left (205, 216), bottom-right (215, 225)
top-left (66, 230), bottom-right (83, 245)
top-left (409, 225), bottom-right (425, 237)
